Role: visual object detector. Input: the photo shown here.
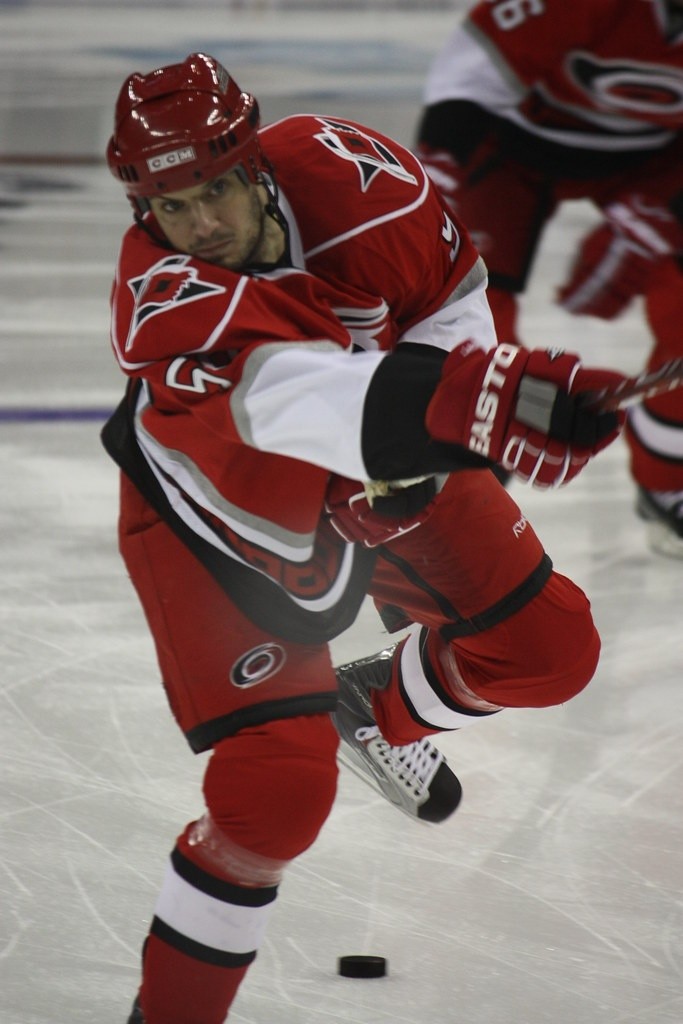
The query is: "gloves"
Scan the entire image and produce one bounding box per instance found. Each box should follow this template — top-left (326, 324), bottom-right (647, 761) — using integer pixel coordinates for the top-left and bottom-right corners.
top-left (426, 342), bottom-right (639, 489)
top-left (558, 220), bottom-right (671, 319)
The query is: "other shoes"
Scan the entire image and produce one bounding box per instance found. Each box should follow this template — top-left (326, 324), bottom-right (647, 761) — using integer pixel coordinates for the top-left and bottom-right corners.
top-left (635, 487), bottom-right (683, 557)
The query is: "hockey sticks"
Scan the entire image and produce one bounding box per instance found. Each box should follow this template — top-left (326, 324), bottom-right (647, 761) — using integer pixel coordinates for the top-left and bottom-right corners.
top-left (574, 356), bottom-right (683, 417)
top-left (0, 406), bottom-right (115, 426)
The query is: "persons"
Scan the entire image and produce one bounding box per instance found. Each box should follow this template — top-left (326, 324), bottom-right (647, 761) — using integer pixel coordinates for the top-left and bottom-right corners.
top-left (372, 0), bottom-right (683, 638)
top-left (94, 43), bottom-right (638, 1024)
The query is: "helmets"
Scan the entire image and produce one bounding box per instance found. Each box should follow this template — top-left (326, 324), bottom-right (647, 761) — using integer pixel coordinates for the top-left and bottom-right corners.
top-left (107, 52), bottom-right (267, 217)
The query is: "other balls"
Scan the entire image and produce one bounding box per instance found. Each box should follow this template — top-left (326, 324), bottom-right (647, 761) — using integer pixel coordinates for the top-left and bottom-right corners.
top-left (338, 955), bottom-right (386, 977)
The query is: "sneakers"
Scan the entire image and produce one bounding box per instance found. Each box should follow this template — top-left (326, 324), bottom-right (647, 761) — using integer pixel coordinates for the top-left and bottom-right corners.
top-left (334, 645), bottom-right (463, 823)
